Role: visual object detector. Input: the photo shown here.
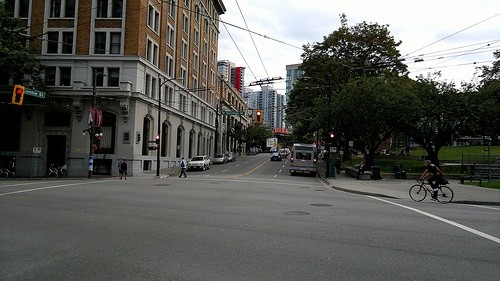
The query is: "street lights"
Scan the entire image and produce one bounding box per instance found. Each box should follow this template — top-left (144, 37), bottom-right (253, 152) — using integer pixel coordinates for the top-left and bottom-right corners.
top-left (156, 77), bottom-right (185, 177)
top-left (83, 72), bottom-right (108, 178)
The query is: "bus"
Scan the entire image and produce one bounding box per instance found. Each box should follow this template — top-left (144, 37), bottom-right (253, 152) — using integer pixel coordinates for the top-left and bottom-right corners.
top-left (266, 138), bottom-right (278, 152)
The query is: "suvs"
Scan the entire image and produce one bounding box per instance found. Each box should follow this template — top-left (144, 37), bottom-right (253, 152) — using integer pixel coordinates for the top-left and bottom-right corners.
top-left (187, 155), bottom-right (211, 171)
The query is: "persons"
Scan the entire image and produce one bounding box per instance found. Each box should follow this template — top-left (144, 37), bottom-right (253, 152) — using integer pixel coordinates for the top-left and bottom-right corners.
top-left (118, 158), bottom-right (127, 180)
top-left (420, 160), bottom-right (443, 201)
top-left (178, 157), bottom-right (187, 178)
top-left (334, 147), bottom-right (411, 180)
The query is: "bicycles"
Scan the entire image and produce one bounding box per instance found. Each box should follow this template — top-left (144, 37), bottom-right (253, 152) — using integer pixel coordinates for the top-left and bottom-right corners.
top-left (409, 178), bottom-right (454, 204)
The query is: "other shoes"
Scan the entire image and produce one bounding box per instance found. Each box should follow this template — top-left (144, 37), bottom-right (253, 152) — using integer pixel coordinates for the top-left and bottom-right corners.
top-left (120, 177), bottom-right (122, 179)
top-left (125, 178), bottom-right (126, 180)
top-left (430, 198), bottom-right (436, 201)
top-left (433, 188), bottom-right (439, 191)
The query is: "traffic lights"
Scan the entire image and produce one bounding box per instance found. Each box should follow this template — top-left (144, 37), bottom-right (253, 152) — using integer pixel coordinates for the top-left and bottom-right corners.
top-left (329, 133), bottom-right (336, 140)
top-left (11, 84), bottom-right (25, 106)
top-left (255, 110), bottom-right (262, 124)
top-left (156, 135), bottom-right (160, 145)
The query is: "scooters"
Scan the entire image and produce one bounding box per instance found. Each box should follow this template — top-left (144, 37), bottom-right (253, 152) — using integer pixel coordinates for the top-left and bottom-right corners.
top-left (46, 161), bottom-right (68, 178)
top-left (0, 165), bottom-right (16, 178)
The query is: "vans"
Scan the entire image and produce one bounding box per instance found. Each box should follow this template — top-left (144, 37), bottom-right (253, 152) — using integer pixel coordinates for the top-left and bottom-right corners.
top-left (289, 143), bottom-right (318, 178)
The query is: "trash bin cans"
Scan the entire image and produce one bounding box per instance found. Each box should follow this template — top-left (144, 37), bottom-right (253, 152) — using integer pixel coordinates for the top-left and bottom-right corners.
top-left (460, 165), bottom-right (476, 180)
top-left (371, 166), bottom-right (381, 180)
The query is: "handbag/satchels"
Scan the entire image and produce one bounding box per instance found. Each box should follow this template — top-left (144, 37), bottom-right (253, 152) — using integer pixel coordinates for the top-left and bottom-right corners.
top-left (181, 160), bottom-right (185, 167)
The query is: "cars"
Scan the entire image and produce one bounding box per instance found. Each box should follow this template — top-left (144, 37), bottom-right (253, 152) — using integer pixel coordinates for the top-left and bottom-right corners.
top-left (278, 148), bottom-right (291, 158)
top-left (212, 154), bottom-right (228, 164)
top-left (225, 152), bottom-right (236, 162)
top-left (270, 152), bottom-right (282, 161)
